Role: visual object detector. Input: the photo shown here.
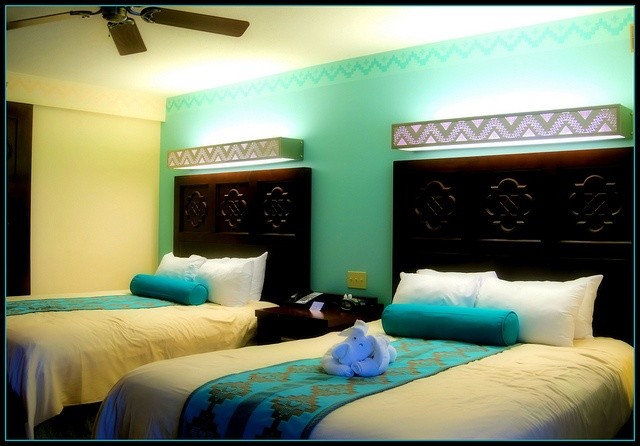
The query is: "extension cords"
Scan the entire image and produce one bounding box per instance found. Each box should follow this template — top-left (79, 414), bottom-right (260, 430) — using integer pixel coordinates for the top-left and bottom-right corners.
top-left (347, 270), bottom-right (367, 290)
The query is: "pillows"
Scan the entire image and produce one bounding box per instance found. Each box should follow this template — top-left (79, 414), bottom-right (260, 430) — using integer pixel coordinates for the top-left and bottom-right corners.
top-left (574, 272), bottom-right (604, 338)
top-left (482, 275), bottom-right (586, 345)
top-left (382, 303), bottom-right (519, 346)
top-left (129, 274), bottom-right (208, 305)
top-left (155, 252), bottom-right (206, 278)
top-left (198, 252), bottom-right (269, 306)
top-left (392, 268), bottom-right (499, 306)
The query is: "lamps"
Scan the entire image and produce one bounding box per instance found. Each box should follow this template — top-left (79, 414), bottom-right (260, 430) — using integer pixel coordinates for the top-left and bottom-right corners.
top-left (391, 104), bottom-right (633, 151)
top-left (167, 137), bottom-right (308, 171)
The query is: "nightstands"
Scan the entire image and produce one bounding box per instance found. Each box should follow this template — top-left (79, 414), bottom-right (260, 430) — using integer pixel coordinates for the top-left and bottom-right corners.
top-left (255, 291), bottom-right (380, 343)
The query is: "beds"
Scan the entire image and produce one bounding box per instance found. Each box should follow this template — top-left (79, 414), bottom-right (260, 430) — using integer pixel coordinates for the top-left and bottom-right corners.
top-left (5, 166), bottom-right (313, 440)
top-left (90, 146), bottom-right (635, 441)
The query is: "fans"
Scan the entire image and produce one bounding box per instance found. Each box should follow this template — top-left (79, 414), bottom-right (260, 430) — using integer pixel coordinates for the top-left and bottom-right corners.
top-left (5, 5), bottom-right (250, 56)
